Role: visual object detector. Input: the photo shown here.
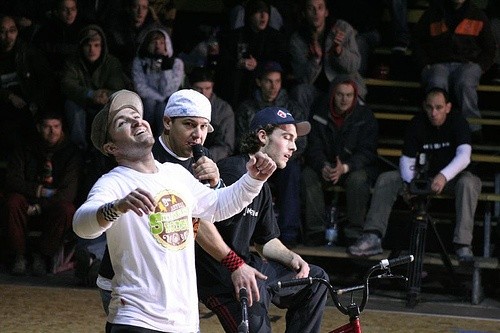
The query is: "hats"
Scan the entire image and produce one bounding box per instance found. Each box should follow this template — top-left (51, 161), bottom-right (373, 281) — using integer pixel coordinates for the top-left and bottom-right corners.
top-left (249, 106), bottom-right (311, 137)
top-left (90, 89), bottom-right (145, 159)
top-left (163, 89), bottom-right (214, 134)
top-left (78, 28), bottom-right (102, 45)
top-left (255, 60), bottom-right (283, 76)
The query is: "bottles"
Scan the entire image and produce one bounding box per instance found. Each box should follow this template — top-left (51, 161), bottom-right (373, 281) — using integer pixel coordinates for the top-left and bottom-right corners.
top-left (206, 30), bottom-right (219, 64)
top-left (324, 207), bottom-right (338, 246)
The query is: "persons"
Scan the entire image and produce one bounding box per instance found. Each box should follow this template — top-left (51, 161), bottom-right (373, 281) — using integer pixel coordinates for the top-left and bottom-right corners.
top-left (346, 88), bottom-right (482, 267)
top-left (71, 89), bottom-right (278, 333)
top-left (190, 107), bottom-right (330, 333)
top-left (96, 88), bottom-right (228, 317)
top-left (0, 0), bottom-right (500, 291)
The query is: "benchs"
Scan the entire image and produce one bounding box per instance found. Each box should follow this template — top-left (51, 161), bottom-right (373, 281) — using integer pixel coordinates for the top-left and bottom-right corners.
top-left (291, 78), bottom-right (500, 304)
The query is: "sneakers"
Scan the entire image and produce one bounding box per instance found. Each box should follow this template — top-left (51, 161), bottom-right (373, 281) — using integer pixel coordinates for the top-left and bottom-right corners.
top-left (346, 232), bottom-right (383, 257)
top-left (455, 244), bottom-right (474, 264)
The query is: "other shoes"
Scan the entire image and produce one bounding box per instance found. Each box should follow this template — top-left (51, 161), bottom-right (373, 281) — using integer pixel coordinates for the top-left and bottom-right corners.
top-left (340, 228), bottom-right (359, 247)
top-left (11, 255), bottom-right (28, 275)
top-left (282, 239), bottom-right (298, 249)
top-left (30, 253), bottom-right (50, 275)
top-left (305, 232), bottom-right (324, 247)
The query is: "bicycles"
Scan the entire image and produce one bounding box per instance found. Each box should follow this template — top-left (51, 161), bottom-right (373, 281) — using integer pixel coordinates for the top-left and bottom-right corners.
top-left (235, 255), bottom-right (414, 333)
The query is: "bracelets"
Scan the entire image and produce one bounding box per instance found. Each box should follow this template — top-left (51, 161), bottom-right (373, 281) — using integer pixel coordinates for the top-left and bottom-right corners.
top-left (220, 250), bottom-right (245, 275)
top-left (101, 200), bottom-right (122, 222)
